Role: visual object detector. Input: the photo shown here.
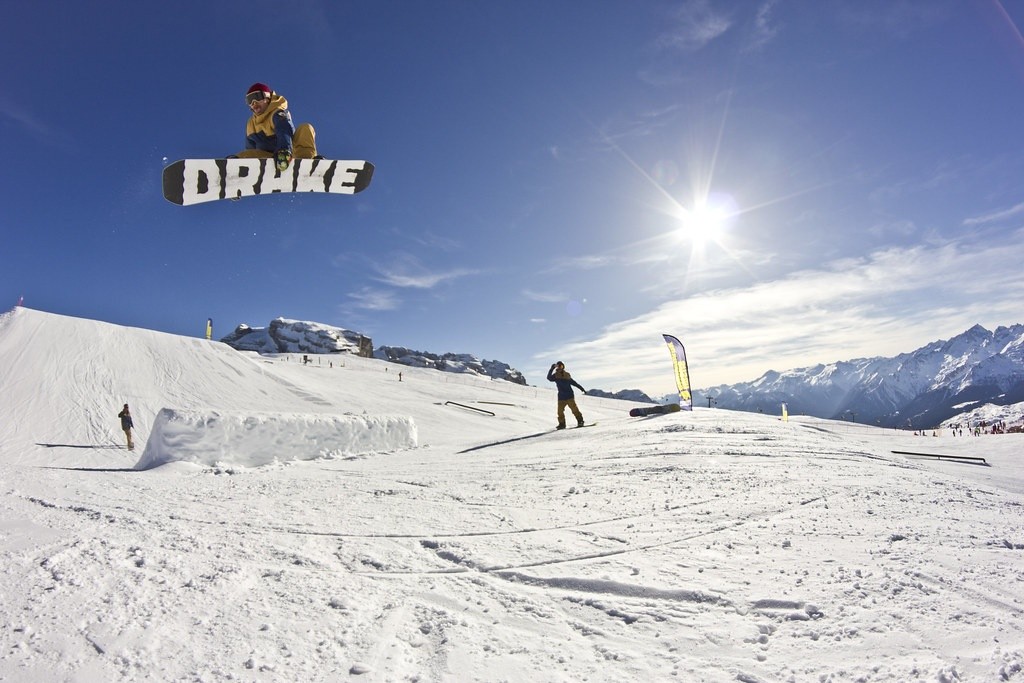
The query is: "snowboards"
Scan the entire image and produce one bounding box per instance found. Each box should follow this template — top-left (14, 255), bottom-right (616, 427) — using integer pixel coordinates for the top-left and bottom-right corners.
top-left (629, 404), bottom-right (681, 417)
top-left (162, 157), bottom-right (375, 207)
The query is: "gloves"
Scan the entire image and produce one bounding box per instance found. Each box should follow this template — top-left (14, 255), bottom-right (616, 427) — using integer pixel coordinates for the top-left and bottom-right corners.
top-left (276, 149), bottom-right (292, 170)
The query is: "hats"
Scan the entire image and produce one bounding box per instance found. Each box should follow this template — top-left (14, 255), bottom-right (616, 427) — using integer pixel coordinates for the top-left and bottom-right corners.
top-left (557, 361), bottom-right (565, 369)
top-left (247, 83), bottom-right (271, 93)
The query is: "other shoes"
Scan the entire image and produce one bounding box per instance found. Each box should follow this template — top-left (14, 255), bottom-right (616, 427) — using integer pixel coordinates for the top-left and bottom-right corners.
top-left (578, 420), bottom-right (584, 427)
top-left (226, 155), bottom-right (238, 158)
top-left (313, 156), bottom-right (326, 160)
top-left (556, 424), bottom-right (566, 428)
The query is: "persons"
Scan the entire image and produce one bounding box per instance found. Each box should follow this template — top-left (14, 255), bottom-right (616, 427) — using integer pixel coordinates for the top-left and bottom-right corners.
top-left (227, 83), bottom-right (325, 170)
top-left (914, 420), bottom-right (1005, 437)
top-left (547, 361), bottom-right (587, 429)
top-left (399, 372), bottom-right (401, 380)
top-left (119, 404), bottom-right (135, 450)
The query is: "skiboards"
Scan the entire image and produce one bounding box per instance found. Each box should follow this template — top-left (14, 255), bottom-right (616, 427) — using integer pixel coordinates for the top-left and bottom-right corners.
top-left (553, 422), bottom-right (596, 432)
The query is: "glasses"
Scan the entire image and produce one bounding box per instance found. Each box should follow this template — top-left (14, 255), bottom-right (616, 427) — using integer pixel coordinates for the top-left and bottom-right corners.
top-left (245, 91), bottom-right (265, 106)
